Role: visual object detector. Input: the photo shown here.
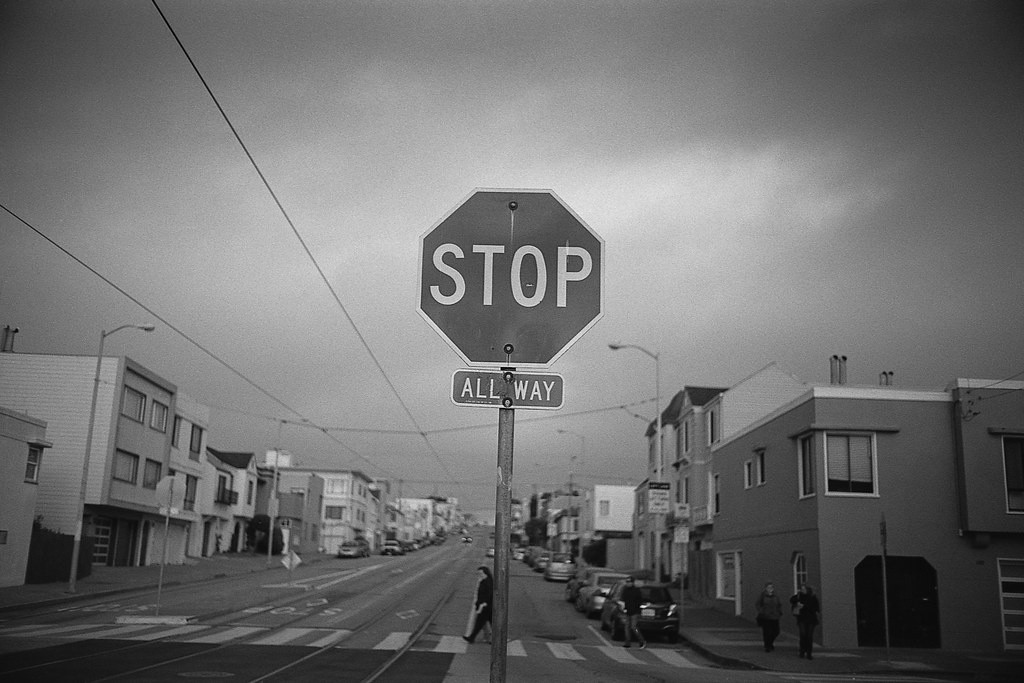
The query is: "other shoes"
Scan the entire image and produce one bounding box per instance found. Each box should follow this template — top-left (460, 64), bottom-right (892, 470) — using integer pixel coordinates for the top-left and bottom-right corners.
top-left (800, 652), bottom-right (804, 658)
top-left (463, 636), bottom-right (473, 643)
top-left (622, 644), bottom-right (631, 649)
top-left (640, 641), bottom-right (646, 650)
top-left (766, 643), bottom-right (774, 652)
top-left (808, 653), bottom-right (812, 659)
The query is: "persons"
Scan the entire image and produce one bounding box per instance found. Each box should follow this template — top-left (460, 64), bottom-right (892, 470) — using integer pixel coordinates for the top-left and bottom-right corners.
top-left (463, 566), bottom-right (493, 644)
top-left (755, 582), bottom-right (784, 651)
top-left (620, 576), bottom-right (647, 649)
top-left (790, 583), bottom-right (819, 659)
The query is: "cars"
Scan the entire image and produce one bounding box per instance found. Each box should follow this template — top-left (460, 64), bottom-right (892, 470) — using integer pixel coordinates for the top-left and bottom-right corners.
top-left (339, 538), bottom-right (370, 558)
top-left (601, 580), bottom-right (680, 645)
top-left (564, 567), bottom-right (634, 618)
top-left (512, 544), bottom-right (576, 582)
top-left (380, 530), bottom-right (448, 556)
top-left (485, 546), bottom-right (495, 557)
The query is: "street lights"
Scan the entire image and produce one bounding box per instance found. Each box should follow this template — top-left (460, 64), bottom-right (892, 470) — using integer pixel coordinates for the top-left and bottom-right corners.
top-left (557, 429), bottom-right (584, 562)
top-left (268, 416), bottom-right (308, 563)
top-left (608, 343), bottom-right (661, 584)
top-left (64, 322), bottom-right (156, 594)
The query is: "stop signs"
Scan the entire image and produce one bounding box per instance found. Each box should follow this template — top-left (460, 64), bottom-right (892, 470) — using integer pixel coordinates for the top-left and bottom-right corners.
top-left (415, 188), bottom-right (605, 368)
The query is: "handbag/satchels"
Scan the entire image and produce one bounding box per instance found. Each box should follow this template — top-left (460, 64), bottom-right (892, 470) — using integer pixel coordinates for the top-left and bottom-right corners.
top-left (793, 593), bottom-right (800, 616)
